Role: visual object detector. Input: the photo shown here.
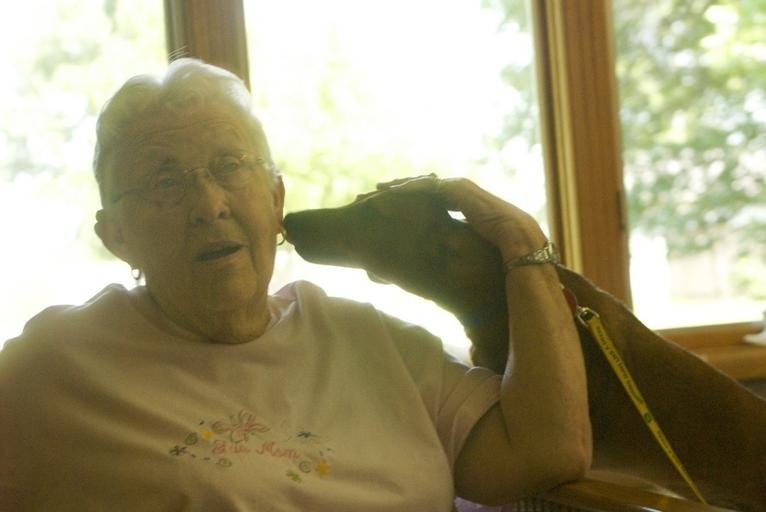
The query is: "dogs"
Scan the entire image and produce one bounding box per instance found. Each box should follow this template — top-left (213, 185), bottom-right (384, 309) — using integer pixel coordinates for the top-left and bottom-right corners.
top-left (282, 174), bottom-right (766, 512)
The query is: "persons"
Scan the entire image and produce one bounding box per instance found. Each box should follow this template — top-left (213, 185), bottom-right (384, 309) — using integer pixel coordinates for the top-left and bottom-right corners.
top-left (0, 57), bottom-right (594, 512)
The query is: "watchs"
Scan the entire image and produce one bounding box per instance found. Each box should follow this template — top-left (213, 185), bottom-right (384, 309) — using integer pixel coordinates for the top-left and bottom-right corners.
top-left (500, 243), bottom-right (556, 271)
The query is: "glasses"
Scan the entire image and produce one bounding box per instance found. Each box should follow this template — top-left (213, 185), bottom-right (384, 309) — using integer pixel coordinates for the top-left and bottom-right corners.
top-left (113, 149), bottom-right (264, 206)
